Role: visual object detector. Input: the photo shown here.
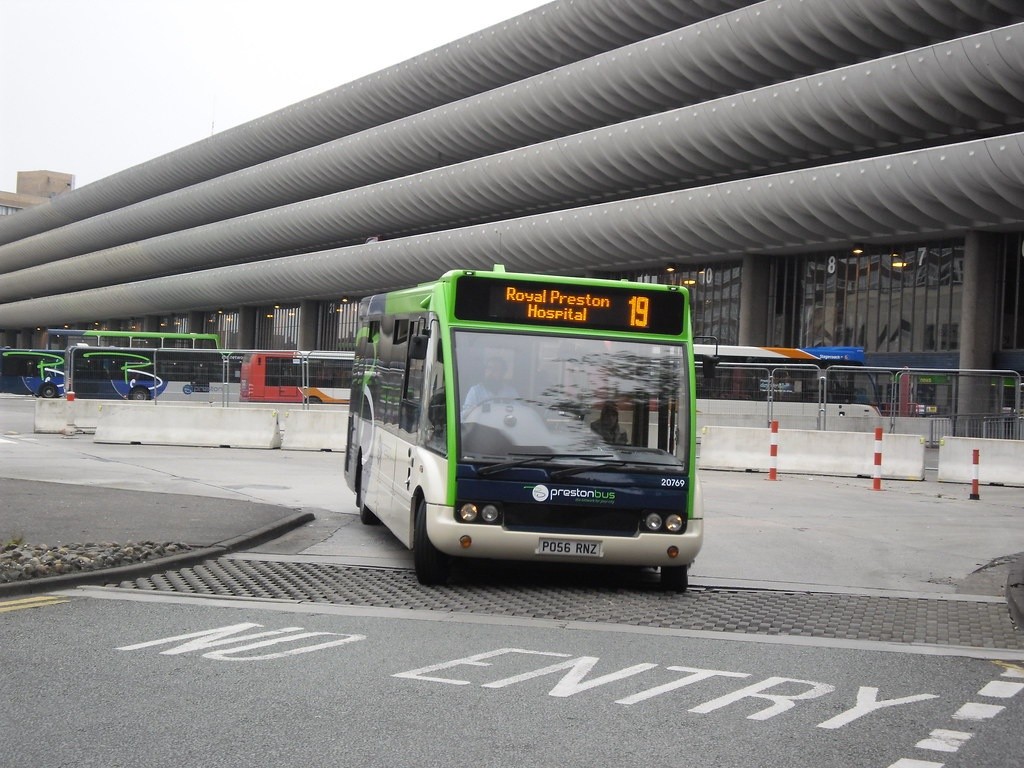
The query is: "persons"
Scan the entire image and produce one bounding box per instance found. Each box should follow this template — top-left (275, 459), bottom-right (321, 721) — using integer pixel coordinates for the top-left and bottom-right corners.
top-left (461, 358), bottom-right (520, 418)
top-left (590, 399), bottom-right (629, 445)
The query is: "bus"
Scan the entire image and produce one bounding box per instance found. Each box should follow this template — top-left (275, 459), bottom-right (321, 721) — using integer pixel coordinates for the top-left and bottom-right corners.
top-left (0, 329), bottom-right (223, 398)
top-left (65, 347), bottom-right (246, 401)
top-left (693, 344), bottom-right (882, 445)
top-left (239, 350), bottom-right (355, 405)
top-left (344, 264), bottom-right (721, 593)
